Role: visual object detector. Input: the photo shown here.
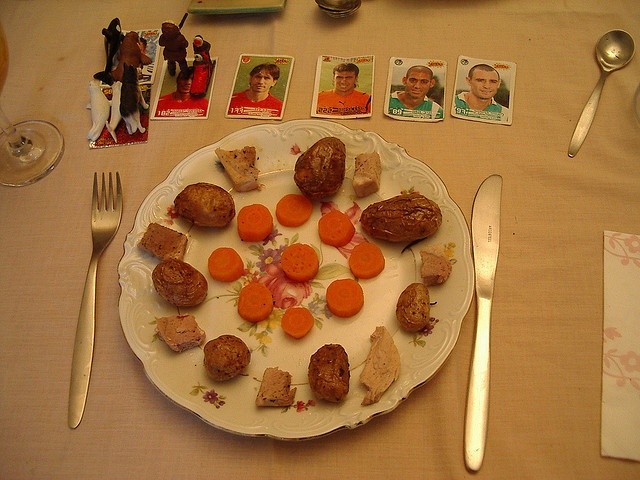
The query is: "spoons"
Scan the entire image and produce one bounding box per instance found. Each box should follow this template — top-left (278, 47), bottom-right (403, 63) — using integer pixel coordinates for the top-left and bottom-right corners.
top-left (567, 29), bottom-right (636, 159)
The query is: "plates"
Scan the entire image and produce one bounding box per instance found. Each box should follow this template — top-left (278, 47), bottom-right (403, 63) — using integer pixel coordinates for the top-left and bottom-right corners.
top-left (116, 119), bottom-right (475, 442)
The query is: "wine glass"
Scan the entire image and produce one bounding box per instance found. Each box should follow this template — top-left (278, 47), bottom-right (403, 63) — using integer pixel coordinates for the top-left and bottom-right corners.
top-left (0, 22), bottom-right (65, 188)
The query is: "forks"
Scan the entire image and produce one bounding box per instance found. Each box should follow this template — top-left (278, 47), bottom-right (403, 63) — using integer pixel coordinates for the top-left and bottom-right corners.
top-left (68, 171), bottom-right (124, 429)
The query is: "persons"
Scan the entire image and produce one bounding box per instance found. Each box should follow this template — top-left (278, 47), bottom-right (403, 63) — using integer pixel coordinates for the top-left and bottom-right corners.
top-left (227, 62), bottom-right (284, 117)
top-left (316, 63), bottom-right (371, 115)
top-left (388, 65), bottom-right (444, 119)
top-left (154, 66), bottom-right (208, 117)
top-left (453, 64), bottom-right (509, 122)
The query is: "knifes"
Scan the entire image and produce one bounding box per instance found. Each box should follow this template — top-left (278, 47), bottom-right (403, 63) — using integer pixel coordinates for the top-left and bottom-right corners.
top-left (463, 174), bottom-right (503, 475)
